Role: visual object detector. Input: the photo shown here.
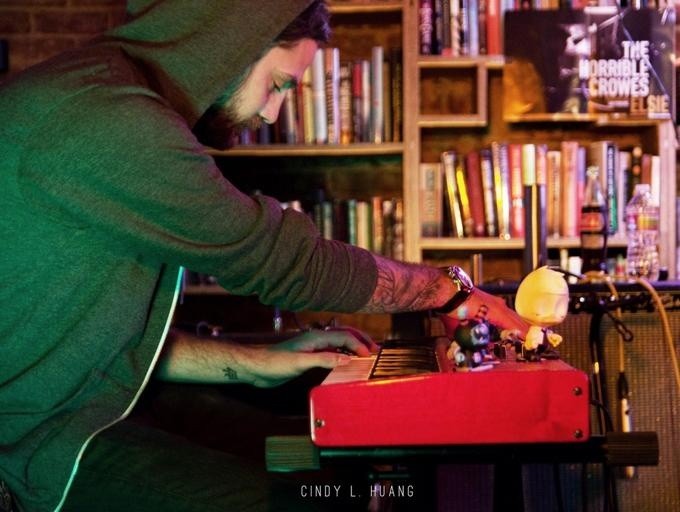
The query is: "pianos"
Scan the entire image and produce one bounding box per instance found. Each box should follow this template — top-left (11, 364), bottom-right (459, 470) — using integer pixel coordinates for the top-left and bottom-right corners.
top-left (307, 315), bottom-right (591, 446)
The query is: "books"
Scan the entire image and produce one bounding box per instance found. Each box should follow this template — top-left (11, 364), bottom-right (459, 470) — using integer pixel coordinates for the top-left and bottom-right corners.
top-left (240, 47), bottom-right (404, 145)
top-left (422, 137), bottom-right (663, 241)
top-left (419, 1), bottom-right (680, 60)
top-left (276, 189), bottom-right (404, 261)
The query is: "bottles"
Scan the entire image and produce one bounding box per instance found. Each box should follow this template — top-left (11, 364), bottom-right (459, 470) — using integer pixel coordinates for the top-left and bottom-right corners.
top-left (580, 167), bottom-right (607, 278)
top-left (626, 183), bottom-right (660, 283)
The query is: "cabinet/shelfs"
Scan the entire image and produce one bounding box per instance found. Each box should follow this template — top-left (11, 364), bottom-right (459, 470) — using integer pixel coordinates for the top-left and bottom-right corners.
top-left (182, 1), bottom-right (408, 339)
top-left (410, 0), bottom-right (678, 290)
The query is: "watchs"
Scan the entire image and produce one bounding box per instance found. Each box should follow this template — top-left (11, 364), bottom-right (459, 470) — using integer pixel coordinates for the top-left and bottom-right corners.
top-left (431, 265), bottom-right (476, 315)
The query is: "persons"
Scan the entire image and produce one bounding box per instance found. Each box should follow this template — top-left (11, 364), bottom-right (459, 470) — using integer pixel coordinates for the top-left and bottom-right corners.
top-left (1, 1), bottom-right (532, 511)
